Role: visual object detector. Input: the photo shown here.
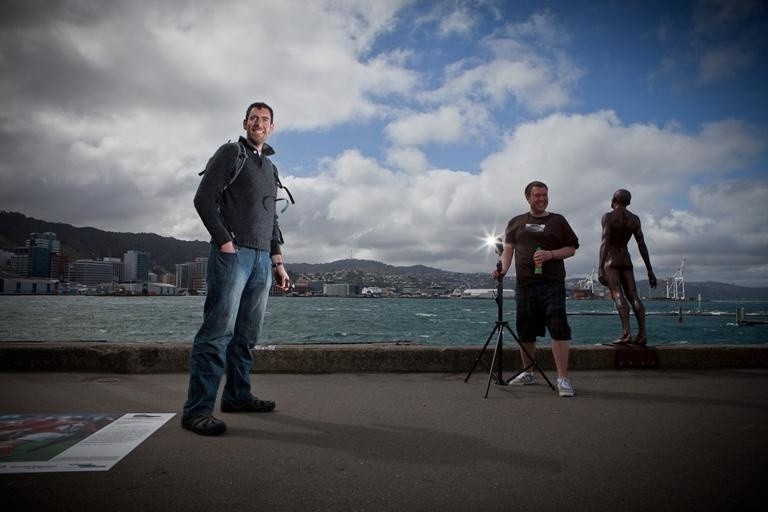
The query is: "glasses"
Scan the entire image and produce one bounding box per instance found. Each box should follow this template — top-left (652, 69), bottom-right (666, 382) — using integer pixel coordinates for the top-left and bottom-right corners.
top-left (272, 278), bottom-right (295, 288)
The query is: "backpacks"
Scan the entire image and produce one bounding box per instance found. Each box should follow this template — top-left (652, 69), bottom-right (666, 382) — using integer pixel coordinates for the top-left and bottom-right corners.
top-left (198, 139), bottom-right (275, 194)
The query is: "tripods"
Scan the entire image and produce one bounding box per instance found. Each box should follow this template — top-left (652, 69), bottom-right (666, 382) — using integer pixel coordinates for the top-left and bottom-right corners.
top-left (464, 255), bottom-right (557, 398)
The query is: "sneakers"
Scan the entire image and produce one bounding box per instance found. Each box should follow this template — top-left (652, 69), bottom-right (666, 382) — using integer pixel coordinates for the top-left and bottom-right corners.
top-left (509, 372), bottom-right (535, 385)
top-left (557, 377), bottom-right (574, 397)
top-left (181, 415), bottom-right (227, 436)
top-left (221, 396), bottom-right (276, 413)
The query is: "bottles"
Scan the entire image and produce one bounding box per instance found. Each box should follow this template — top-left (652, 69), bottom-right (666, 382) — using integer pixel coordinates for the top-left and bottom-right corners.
top-left (534, 246), bottom-right (544, 275)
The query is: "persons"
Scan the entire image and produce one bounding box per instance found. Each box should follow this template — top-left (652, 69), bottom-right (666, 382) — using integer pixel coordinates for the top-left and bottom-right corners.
top-left (181, 102), bottom-right (295, 436)
top-left (490, 181), bottom-right (580, 397)
top-left (597, 189), bottom-right (655, 350)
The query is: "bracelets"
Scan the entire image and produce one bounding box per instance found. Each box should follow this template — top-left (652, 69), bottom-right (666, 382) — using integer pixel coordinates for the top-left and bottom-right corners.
top-left (549, 249), bottom-right (553, 264)
top-left (271, 263), bottom-right (283, 267)
